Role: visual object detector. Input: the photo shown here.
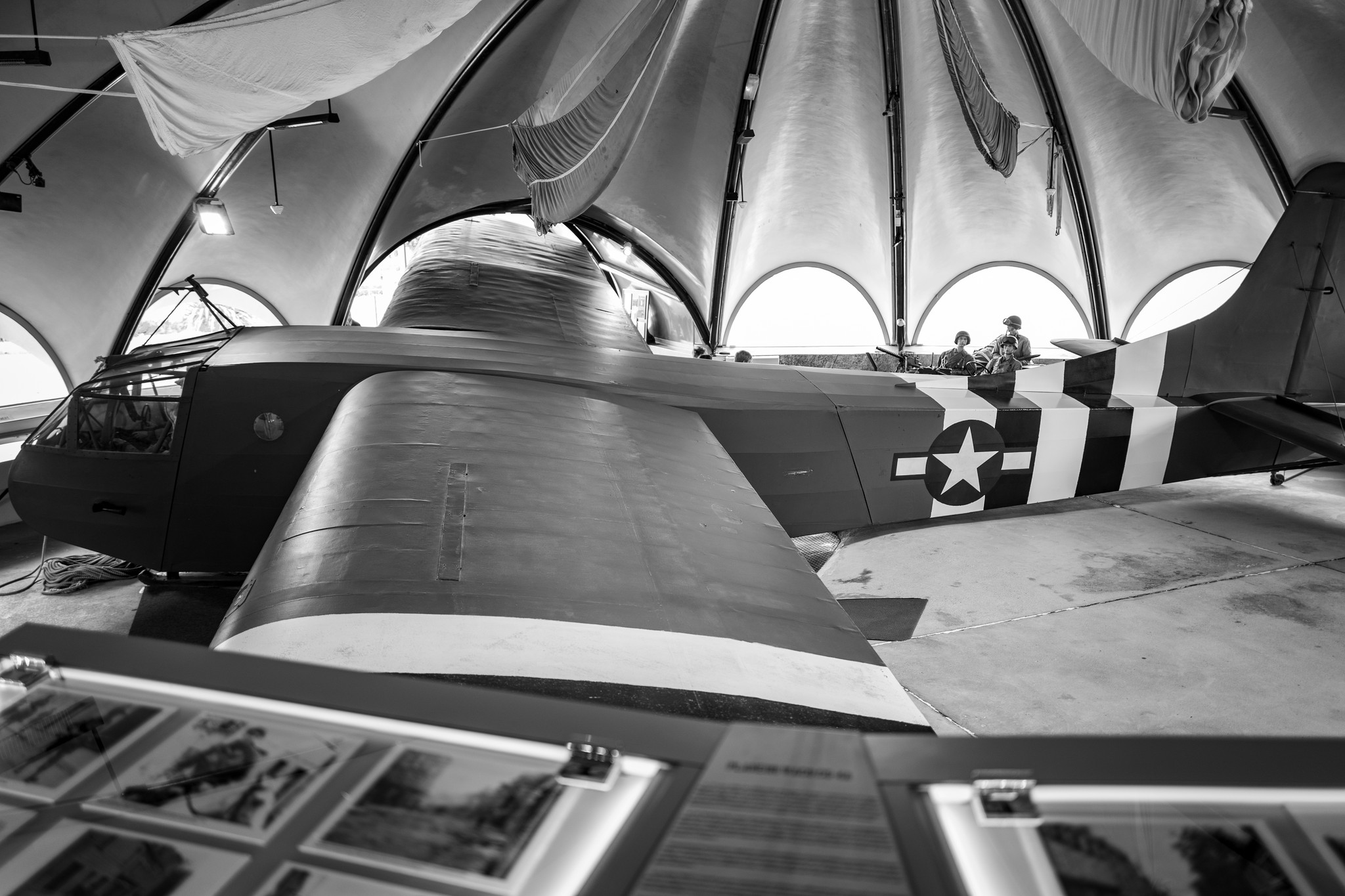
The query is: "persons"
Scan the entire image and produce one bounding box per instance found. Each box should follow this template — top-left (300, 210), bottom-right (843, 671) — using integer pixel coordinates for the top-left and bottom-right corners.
top-left (990, 316), bottom-right (1031, 364)
top-left (980, 335), bottom-right (1022, 375)
top-left (937, 331), bottom-right (977, 376)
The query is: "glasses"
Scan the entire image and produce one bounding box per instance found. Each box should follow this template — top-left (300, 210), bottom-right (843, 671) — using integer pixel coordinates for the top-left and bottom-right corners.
top-left (1001, 337), bottom-right (1018, 345)
top-left (1003, 318), bottom-right (1021, 326)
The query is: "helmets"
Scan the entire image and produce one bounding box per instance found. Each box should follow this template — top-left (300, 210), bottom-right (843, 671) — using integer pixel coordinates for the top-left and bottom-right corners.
top-left (1000, 335), bottom-right (1018, 350)
top-left (954, 331), bottom-right (970, 345)
top-left (1003, 316), bottom-right (1021, 329)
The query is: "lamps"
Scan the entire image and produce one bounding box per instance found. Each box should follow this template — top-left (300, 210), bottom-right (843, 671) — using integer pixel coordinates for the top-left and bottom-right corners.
top-left (191, 197), bottom-right (235, 235)
